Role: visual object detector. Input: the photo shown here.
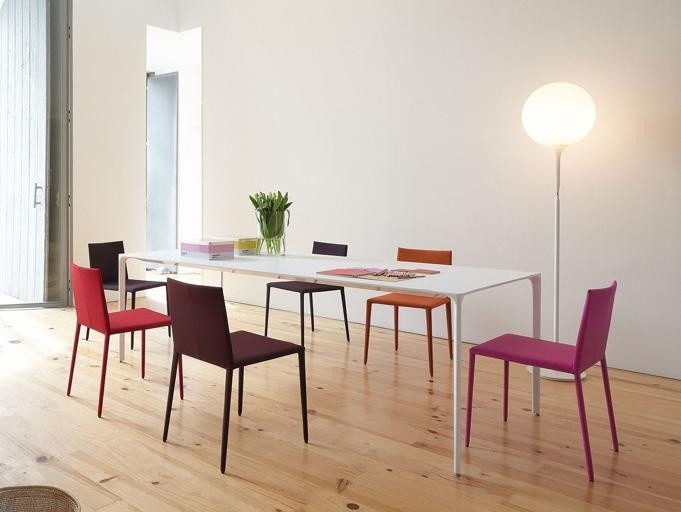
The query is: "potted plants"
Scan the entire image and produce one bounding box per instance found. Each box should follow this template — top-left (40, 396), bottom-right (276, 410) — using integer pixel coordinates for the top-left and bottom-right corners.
top-left (247, 189), bottom-right (294, 257)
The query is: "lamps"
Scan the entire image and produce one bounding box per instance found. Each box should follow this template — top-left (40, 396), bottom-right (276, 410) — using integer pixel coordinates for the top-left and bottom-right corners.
top-left (521, 80), bottom-right (598, 383)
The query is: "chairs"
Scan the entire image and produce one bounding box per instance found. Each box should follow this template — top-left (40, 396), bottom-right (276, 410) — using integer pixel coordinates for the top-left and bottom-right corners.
top-left (264, 241), bottom-right (349, 344)
top-left (66, 259), bottom-right (171, 418)
top-left (161, 277), bottom-right (310, 474)
top-left (88, 241), bottom-right (170, 348)
top-left (461, 279), bottom-right (632, 483)
top-left (361, 247), bottom-right (459, 376)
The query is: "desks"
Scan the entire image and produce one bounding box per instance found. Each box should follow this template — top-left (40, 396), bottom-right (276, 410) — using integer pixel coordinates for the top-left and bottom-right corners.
top-left (118, 249), bottom-right (543, 477)
